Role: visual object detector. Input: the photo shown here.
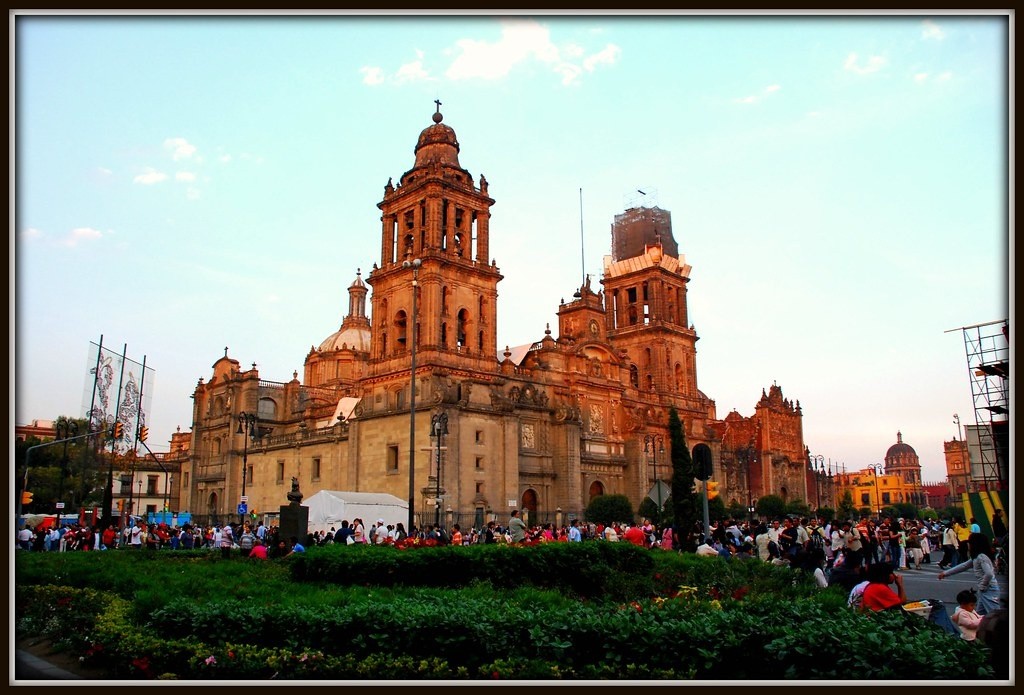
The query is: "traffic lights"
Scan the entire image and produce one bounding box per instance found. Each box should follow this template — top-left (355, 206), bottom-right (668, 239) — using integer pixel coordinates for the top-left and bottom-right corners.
top-left (22, 491), bottom-right (34, 504)
top-left (706, 482), bottom-right (720, 499)
top-left (164, 502), bottom-right (170, 512)
top-left (115, 422), bottom-right (123, 439)
top-left (141, 425), bottom-right (149, 442)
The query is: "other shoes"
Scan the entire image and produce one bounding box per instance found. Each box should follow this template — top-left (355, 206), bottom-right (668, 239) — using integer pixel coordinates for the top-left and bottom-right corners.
top-left (939, 563), bottom-right (944, 569)
top-left (900, 567), bottom-right (909, 570)
top-left (916, 565), bottom-right (921, 570)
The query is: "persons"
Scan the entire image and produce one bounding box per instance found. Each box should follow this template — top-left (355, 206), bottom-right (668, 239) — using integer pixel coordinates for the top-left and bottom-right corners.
top-left (14, 508), bottom-right (1009, 643)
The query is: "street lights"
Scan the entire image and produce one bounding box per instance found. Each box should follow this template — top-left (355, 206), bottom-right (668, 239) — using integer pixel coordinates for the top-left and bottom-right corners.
top-left (403, 258), bottom-right (422, 534)
top-left (54, 419), bottom-right (79, 530)
top-left (868, 463), bottom-right (883, 519)
top-left (428, 412), bottom-right (449, 527)
top-left (807, 454), bottom-right (825, 511)
top-left (951, 474), bottom-right (959, 515)
top-left (737, 444), bottom-right (757, 520)
top-left (170, 476), bottom-right (174, 511)
top-left (643, 432), bottom-right (666, 485)
top-left (136, 479), bottom-right (142, 524)
top-left (237, 410), bottom-right (257, 537)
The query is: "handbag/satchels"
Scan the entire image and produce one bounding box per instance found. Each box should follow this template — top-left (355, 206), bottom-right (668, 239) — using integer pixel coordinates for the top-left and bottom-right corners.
top-left (346, 535), bottom-right (355, 546)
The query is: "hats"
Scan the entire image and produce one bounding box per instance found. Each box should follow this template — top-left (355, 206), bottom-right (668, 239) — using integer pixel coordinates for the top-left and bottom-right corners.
top-left (159, 523), bottom-right (166, 527)
top-left (377, 519), bottom-right (384, 524)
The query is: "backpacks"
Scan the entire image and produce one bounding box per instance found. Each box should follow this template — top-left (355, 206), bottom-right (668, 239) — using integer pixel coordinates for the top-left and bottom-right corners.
top-left (810, 527), bottom-right (821, 542)
